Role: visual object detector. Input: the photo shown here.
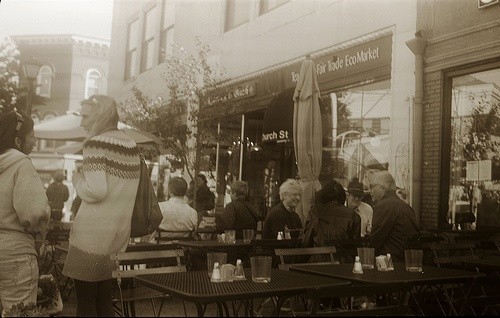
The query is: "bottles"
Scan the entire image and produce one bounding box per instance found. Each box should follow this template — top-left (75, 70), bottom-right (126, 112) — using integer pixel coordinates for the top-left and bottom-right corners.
top-left (210, 262), bottom-right (224, 282)
top-left (353, 256), bottom-right (363, 274)
top-left (386, 253), bottom-right (394, 270)
top-left (277, 228), bottom-right (292, 240)
top-left (233, 259), bottom-right (247, 280)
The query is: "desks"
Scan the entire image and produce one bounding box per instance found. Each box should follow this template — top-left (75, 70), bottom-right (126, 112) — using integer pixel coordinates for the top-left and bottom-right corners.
top-left (56, 229), bottom-right (500, 318)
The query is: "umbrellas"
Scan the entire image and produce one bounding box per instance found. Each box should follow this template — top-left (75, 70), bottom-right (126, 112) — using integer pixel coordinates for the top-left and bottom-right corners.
top-left (293, 52), bottom-right (324, 226)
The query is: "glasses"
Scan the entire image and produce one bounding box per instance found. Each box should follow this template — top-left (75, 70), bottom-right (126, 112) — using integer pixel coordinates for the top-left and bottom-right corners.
top-left (350, 193), bottom-right (363, 199)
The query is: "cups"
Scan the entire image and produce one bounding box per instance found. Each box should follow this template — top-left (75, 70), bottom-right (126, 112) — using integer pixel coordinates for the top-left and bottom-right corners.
top-left (243, 230), bottom-right (254, 244)
top-left (250, 256), bottom-right (272, 283)
top-left (207, 252), bottom-right (227, 278)
top-left (405, 249), bottom-right (425, 272)
top-left (358, 247), bottom-right (375, 269)
top-left (225, 229), bottom-right (237, 244)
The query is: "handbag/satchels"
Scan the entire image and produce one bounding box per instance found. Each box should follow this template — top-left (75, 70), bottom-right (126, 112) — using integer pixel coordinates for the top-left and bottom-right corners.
top-left (408, 286), bottom-right (450, 317)
top-left (129, 152), bottom-right (163, 238)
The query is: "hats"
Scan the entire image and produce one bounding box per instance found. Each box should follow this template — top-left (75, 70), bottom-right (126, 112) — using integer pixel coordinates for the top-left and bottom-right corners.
top-left (345, 183), bottom-right (364, 192)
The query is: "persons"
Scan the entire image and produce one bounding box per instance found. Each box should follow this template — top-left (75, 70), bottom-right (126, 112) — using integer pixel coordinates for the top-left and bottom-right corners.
top-left (63, 95), bottom-right (141, 317)
top-left (154, 176), bottom-right (197, 243)
top-left (45, 171), bottom-right (70, 221)
top-left (260, 178), bottom-right (305, 242)
top-left (365, 170), bottom-right (421, 262)
top-left (187, 173), bottom-right (215, 228)
top-left (218, 180), bottom-right (257, 237)
top-left (343, 176), bottom-right (373, 239)
top-left (303, 180), bottom-right (362, 249)
top-left (0, 110), bottom-right (51, 317)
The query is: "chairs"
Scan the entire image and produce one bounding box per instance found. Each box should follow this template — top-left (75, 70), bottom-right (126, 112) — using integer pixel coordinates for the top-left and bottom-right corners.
top-left (154, 228), bottom-right (196, 267)
top-left (427, 240), bottom-right (500, 318)
top-left (42, 227), bottom-right (75, 301)
top-left (274, 244), bottom-right (341, 311)
top-left (107, 249), bottom-right (190, 318)
top-left (249, 239), bottom-right (311, 311)
